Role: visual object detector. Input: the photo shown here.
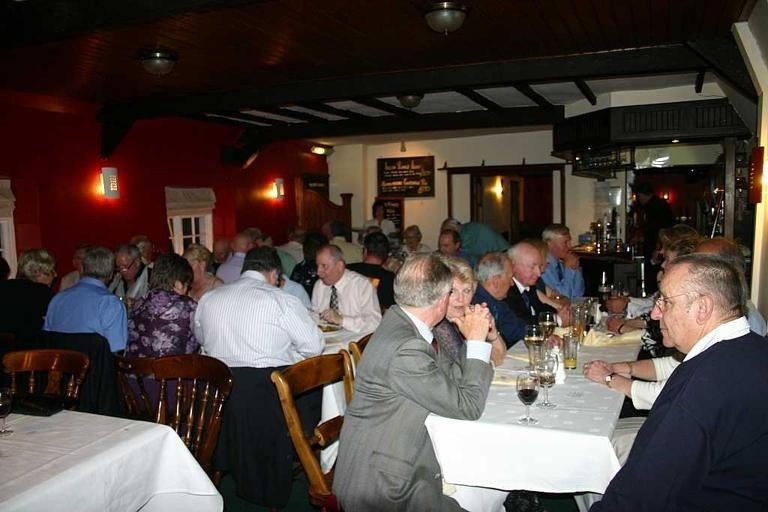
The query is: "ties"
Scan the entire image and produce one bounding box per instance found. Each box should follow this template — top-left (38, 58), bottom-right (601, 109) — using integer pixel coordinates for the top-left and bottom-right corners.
top-left (522, 290), bottom-right (531, 321)
top-left (329, 286), bottom-right (339, 314)
top-left (557, 263), bottom-right (563, 281)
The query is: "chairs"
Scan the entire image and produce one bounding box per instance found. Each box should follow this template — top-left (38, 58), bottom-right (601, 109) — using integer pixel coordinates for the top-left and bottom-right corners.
top-left (349, 332), bottom-right (374, 365)
top-left (4, 348), bottom-right (90, 407)
top-left (268, 348), bottom-right (361, 511)
top-left (148, 356), bottom-right (234, 467)
top-left (113, 348), bottom-right (165, 421)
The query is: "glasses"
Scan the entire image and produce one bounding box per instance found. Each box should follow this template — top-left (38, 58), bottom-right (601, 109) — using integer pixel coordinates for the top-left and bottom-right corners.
top-left (653, 292), bottom-right (688, 313)
top-left (115, 260), bottom-right (136, 273)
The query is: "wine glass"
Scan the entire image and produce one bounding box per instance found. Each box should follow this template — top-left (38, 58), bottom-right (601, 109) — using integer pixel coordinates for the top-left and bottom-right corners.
top-left (517, 311), bottom-right (558, 426)
top-left (0, 387), bottom-right (14, 437)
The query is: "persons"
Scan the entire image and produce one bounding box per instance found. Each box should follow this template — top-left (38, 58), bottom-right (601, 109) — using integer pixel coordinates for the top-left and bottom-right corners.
top-left (0, 185), bottom-right (767, 512)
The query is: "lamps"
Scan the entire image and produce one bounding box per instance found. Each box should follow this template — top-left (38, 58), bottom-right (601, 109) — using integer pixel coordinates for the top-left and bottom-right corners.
top-left (272, 176), bottom-right (285, 199)
top-left (141, 50), bottom-right (177, 76)
top-left (396, 92), bottom-right (424, 108)
top-left (99, 166), bottom-right (120, 198)
top-left (425, 3), bottom-right (467, 34)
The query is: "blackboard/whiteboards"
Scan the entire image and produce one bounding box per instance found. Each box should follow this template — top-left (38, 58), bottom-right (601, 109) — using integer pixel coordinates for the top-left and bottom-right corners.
top-left (375, 196), bottom-right (404, 240)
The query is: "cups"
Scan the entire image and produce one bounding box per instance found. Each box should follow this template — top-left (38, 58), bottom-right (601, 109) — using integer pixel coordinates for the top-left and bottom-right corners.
top-left (563, 296), bottom-right (598, 370)
